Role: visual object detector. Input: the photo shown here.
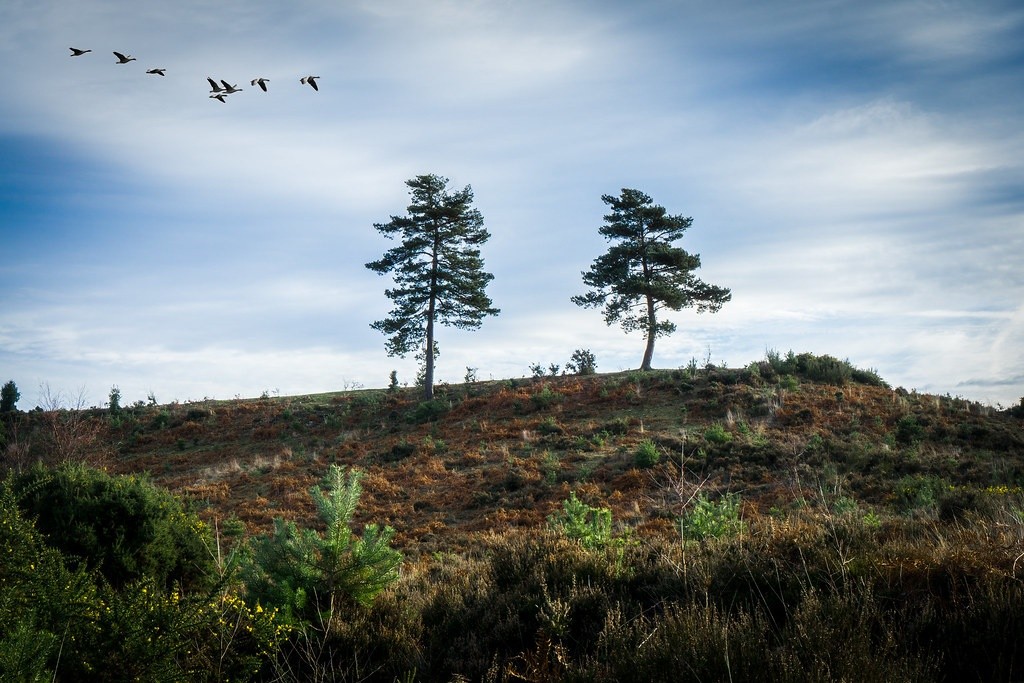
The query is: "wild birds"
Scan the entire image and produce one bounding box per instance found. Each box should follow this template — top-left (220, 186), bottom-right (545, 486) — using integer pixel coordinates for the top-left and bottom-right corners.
top-left (249, 78), bottom-right (270, 92)
top-left (69, 48), bottom-right (92, 57)
top-left (112, 52), bottom-right (136, 64)
top-left (145, 68), bottom-right (166, 76)
top-left (299, 76), bottom-right (320, 91)
top-left (206, 76), bottom-right (243, 103)
